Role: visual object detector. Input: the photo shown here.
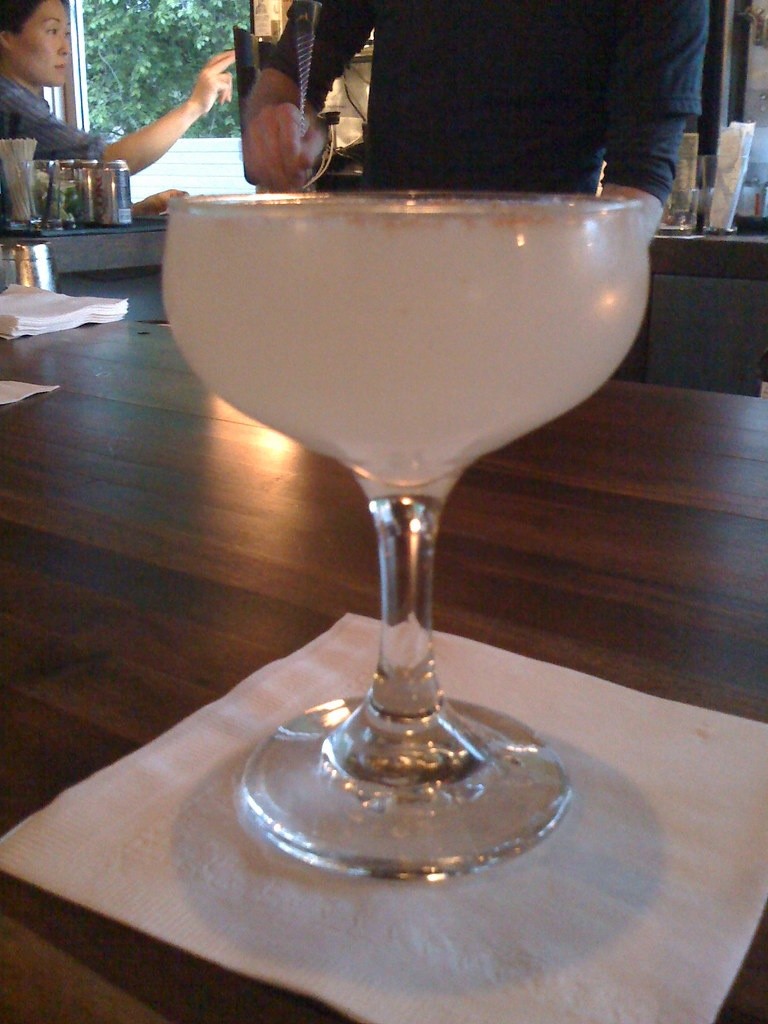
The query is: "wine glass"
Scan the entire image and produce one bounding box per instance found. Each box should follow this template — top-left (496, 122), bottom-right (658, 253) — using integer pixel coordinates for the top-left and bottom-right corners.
top-left (162, 188), bottom-right (668, 885)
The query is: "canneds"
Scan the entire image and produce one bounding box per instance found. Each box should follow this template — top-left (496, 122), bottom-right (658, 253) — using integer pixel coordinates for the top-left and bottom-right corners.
top-left (37, 159), bottom-right (134, 226)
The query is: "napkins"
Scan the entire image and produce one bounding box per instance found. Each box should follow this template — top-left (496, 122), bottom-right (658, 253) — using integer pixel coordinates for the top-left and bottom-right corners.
top-left (1, 610), bottom-right (768, 1024)
top-left (0, 379), bottom-right (61, 405)
top-left (0, 285), bottom-right (130, 339)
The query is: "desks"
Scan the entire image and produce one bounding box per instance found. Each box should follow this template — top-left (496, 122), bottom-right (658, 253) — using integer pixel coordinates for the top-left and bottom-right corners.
top-left (0, 324), bottom-right (768, 1024)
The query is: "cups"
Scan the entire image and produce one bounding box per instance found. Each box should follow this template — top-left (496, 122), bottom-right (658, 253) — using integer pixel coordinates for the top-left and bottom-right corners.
top-left (0, 239), bottom-right (58, 297)
top-left (0, 157), bottom-right (63, 231)
top-left (654, 155), bottom-right (749, 236)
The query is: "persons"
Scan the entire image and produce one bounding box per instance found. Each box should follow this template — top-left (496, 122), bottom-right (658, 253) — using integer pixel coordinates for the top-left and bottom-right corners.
top-left (0, 0), bottom-right (237, 218)
top-left (242, 0), bottom-right (711, 236)
